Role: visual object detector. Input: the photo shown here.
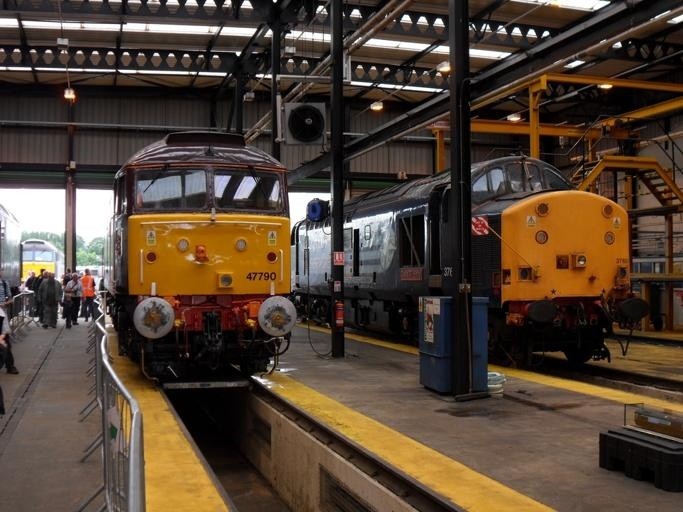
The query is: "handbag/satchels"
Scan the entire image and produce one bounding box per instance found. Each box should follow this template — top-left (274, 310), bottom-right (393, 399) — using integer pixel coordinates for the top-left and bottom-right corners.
top-left (64, 292), bottom-right (72, 303)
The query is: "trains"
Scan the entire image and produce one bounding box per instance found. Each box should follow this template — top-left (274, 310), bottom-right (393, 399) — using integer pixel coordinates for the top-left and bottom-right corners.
top-left (287, 149), bottom-right (650, 374)
top-left (0, 201), bottom-right (24, 308)
top-left (17, 236), bottom-right (65, 288)
top-left (95, 125), bottom-right (303, 399)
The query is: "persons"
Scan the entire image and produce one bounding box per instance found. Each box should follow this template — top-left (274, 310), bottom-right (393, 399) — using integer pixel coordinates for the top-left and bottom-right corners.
top-left (0, 385), bottom-right (8, 422)
top-left (0, 306), bottom-right (20, 374)
top-left (26, 268), bottom-right (96, 330)
top-left (0, 269), bottom-right (21, 327)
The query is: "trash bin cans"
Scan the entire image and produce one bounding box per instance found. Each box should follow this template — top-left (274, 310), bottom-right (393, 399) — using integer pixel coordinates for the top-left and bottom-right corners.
top-left (418, 297), bottom-right (490, 395)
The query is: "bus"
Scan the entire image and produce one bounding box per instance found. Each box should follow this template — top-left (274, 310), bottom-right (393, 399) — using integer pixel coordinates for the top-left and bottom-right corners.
top-left (74, 265), bottom-right (98, 291)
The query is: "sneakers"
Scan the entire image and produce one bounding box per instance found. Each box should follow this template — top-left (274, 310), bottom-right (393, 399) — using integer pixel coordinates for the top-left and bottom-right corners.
top-left (42, 322), bottom-right (79, 329)
top-left (5, 366), bottom-right (19, 375)
